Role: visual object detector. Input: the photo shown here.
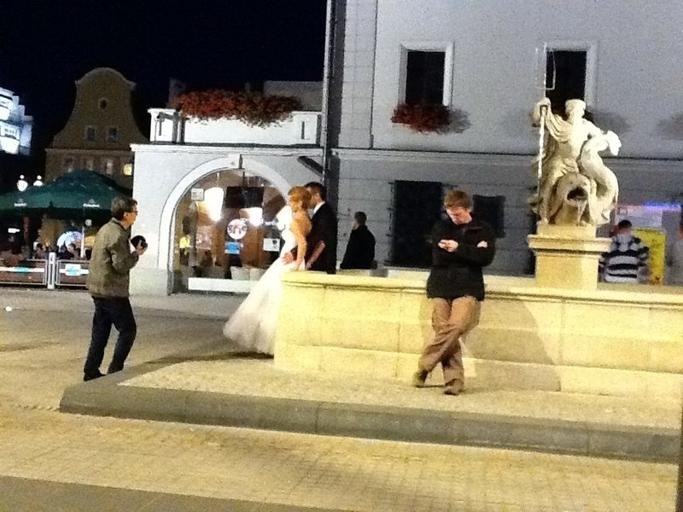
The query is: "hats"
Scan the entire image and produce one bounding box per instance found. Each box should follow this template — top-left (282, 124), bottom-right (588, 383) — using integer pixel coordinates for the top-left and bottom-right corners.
top-left (618, 220), bottom-right (632, 229)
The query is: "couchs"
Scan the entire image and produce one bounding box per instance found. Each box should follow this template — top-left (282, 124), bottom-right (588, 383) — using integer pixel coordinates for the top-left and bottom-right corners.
top-left (173, 260), bottom-right (266, 294)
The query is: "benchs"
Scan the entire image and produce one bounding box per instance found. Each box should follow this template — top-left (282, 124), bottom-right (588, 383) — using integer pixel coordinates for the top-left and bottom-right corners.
top-left (0, 257), bottom-right (91, 285)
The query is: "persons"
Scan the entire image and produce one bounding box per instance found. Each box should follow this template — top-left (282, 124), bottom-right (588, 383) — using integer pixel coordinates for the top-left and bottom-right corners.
top-left (282, 182), bottom-right (337, 275)
top-left (597, 220), bottom-right (649, 284)
top-left (196, 249), bottom-right (212, 276)
top-left (665, 220), bottom-right (682, 285)
top-left (340, 212), bottom-right (374, 270)
top-left (531, 96), bottom-right (605, 227)
top-left (223, 253), bottom-right (243, 279)
top-left (411, 192), bottom-right (494, 395)
top-left (222, 187), bottom-right (313, 356)
top-left (3, 241), bottom-right (81, 274)
top-left (83, 198), bottom-right (146, 382)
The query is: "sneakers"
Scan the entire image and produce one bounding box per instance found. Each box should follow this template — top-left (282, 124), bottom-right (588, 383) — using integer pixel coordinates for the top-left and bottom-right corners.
top-left (444, 379), bottom-right (464, 395)
top-left (412, 369), bottom-right (427, 388)
top-left (84, 370), bottom-right (105, 381)
